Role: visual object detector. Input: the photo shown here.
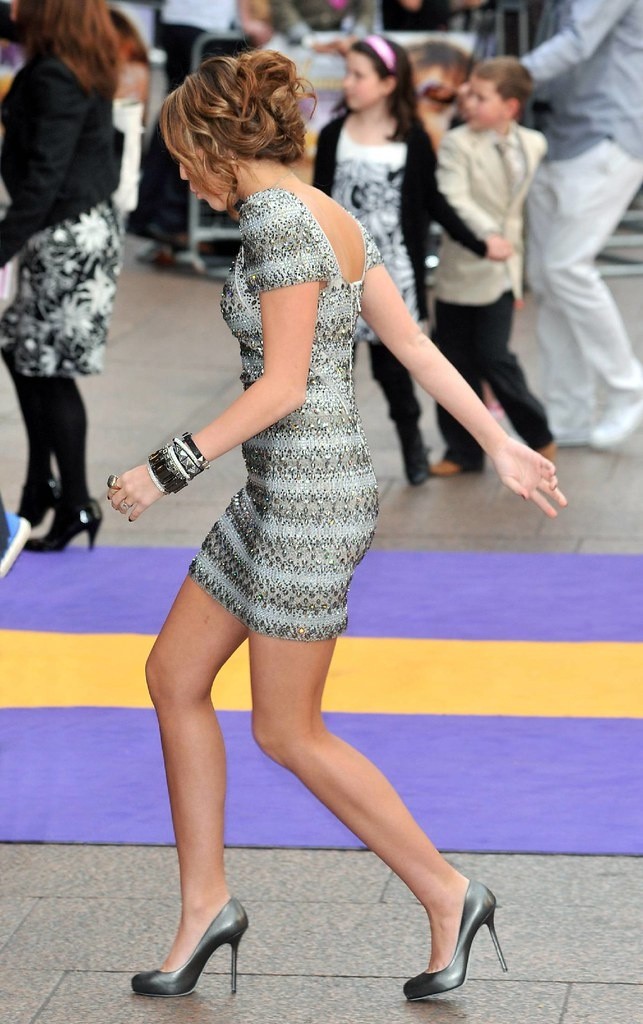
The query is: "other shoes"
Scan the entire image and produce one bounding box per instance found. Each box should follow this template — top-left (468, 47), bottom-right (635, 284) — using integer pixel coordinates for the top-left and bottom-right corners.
top-left (551, 428), bottom-right (592, 447)
top-left (398, 424), bottom-right (428, 485)
top-left (424, 460), bottom-right (461, 476)
top-left (590, 397), bottom-right (643, 449)
top-left (536, 443), bottom-right (556, 464)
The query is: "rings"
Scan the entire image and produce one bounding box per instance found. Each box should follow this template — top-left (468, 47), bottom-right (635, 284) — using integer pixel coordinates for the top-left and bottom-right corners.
top-left (107, 475), bottom-right (119, 488)
top-left (122, 500), bottom-right (132, 509)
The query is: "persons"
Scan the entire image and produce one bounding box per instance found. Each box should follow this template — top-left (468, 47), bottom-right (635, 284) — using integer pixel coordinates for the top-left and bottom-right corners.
top-left (431, 56), bottom-right (557, 474)
top-left (0, 0), bottom-right (120, 551)
top-left (313, 35), bottom-right (513, 483)
top-left (102, 50), bottom-right (569, 998)
top-left (104, 0), bottom-right (455, 271)
top-left (512, 0), bottom-right (643, 450)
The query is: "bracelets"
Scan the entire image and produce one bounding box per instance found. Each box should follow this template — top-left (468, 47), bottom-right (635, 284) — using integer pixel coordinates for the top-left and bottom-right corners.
top-left (144, 431), bottom-right (211, 495)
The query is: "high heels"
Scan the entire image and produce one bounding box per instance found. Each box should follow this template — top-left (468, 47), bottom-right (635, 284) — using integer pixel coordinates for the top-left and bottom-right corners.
top-left (130, 898), bottom-right (248, 998)
top-left (404, 879), bottom-right (508, 999)
top-left (21, 500), bottom-right (103, 552)
top-left (19, 479), bottom-right (62, 526)
